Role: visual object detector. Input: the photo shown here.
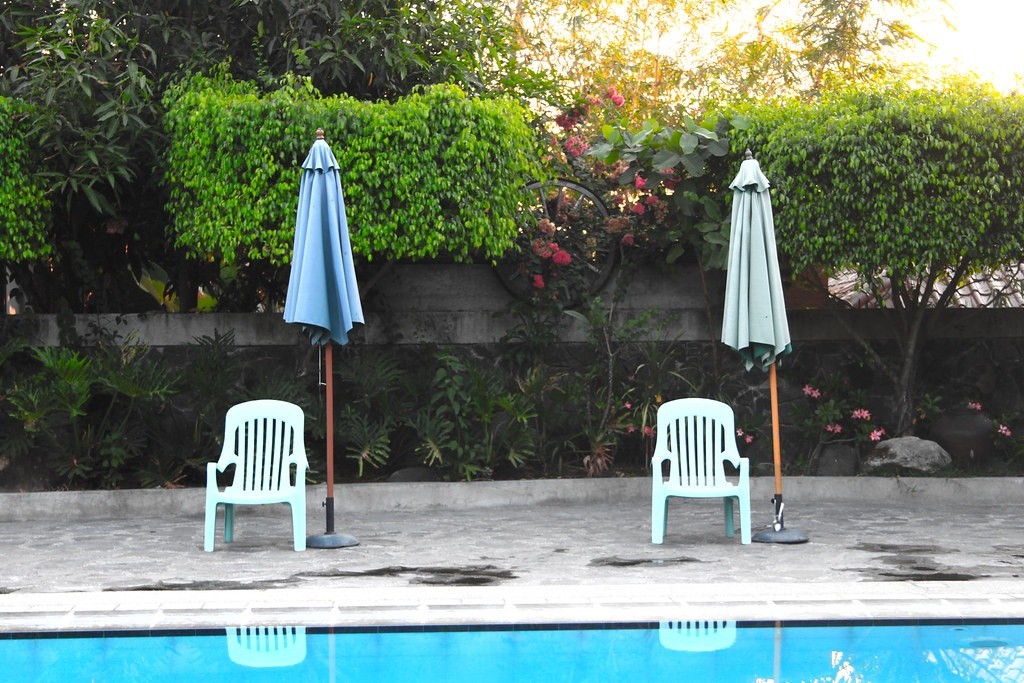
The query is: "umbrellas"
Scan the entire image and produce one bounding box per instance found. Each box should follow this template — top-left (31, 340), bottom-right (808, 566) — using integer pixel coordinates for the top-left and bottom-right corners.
top-left (722, 148), bottom-right (810, 544)
top-left (284, 127), bottom-right (365, 548)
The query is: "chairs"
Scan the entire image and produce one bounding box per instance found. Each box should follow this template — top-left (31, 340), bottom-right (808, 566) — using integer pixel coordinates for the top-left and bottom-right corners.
top-left (652, 398), bottom-right (752, 545)
top-left (660, 620), bottom-right (738, 653)
top-left (205, 400), bottom-right (309, 551)
top-left (225, 626), bottom-right (307, 666)
